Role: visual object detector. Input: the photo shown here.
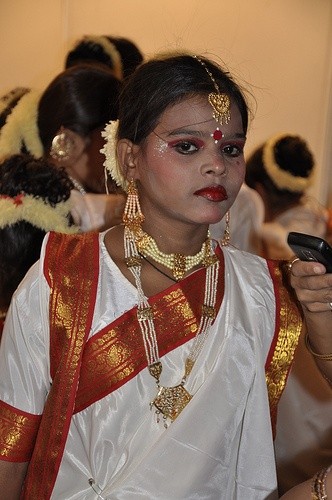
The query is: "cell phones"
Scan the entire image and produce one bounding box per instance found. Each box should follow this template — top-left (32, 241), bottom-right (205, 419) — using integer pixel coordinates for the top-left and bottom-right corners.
top-left (286, 231), bottom-right (332, 275)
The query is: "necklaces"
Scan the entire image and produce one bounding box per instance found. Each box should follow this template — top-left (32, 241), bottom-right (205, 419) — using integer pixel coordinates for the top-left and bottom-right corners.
top-left (135, 227), bottom-right (205, 280)
top-left (124, 224), bottom-right (220, 429)
top-left (69, 176), bottom-right (85, 194)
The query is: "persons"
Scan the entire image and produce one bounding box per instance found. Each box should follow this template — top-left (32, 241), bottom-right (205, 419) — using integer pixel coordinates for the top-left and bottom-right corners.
top-left (0, 35), bottom-right (332, 499)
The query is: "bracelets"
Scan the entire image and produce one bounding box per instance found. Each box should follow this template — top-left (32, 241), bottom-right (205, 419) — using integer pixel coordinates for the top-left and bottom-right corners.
top-left (311, 465), bottom-right (332, 500)
top-left (305, 334), bottom-right (332, 360)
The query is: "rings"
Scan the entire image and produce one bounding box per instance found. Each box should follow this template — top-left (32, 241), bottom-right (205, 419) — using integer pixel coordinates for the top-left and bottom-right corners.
top-left (328, 303), bottom-right (332, 309)
top-left (287, 257), bottom-right (300, 277)
